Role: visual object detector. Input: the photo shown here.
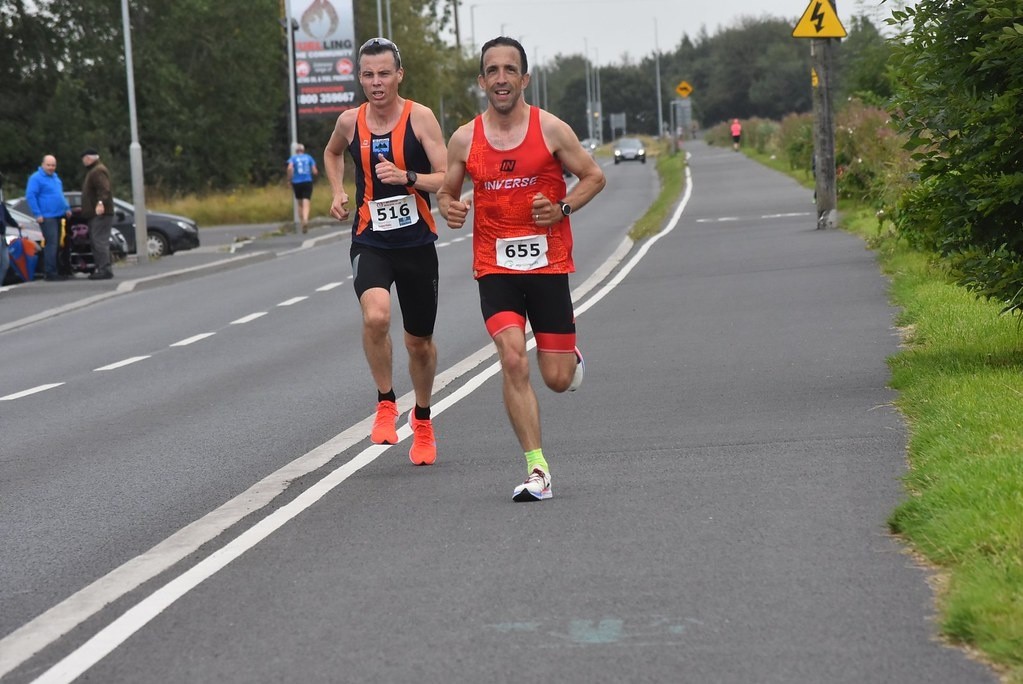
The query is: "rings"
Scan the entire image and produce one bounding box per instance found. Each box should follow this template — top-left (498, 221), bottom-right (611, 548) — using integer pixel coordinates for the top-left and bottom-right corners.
top-left (536, 215), bottom-right (539, 221)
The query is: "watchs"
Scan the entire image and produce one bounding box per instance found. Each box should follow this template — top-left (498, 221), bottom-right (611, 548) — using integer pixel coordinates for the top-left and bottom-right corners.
top-left (406, 170), bottom-right (416, 187)
top-left (558, 201), bottom-right (572, 216)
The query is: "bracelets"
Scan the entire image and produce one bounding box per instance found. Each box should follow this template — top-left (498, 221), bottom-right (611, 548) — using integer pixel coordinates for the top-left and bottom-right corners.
top-left (99, 201), bottom-right (102, 204)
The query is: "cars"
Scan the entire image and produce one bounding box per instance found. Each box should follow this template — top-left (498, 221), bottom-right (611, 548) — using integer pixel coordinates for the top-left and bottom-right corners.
top-left (8, 191), bottom-right (201, 258)
top-left (4, 202), bottom-right (128, 274)
top-left (561, 137), bottom-right (600, 177)
top-left (612, 138), bottom-right (647, 163)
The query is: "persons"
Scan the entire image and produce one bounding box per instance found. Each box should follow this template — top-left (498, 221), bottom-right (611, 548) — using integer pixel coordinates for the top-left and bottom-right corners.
top-left (730, 118), bottom-right (742, 151)
top-left (692, 125), bottom-right (696, 139)
top-left (324, 39), bottom-right (448, 465)
top-left (436, 36), bottom-right (605, 503)
top-left (79, 150), bottom-right (114, 280)
top-left (287, 143), bottom-right (317, 233)
top-left (677, 125), bottom-right (683, 139)
top-left (25, 155), bottom-right (72, 281)
top-left (0, 200), bottom-right (25, 286)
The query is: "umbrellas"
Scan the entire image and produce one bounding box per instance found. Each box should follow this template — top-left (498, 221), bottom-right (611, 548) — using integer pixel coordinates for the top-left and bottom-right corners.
top-left (8, 228), bottom-right (41, 282)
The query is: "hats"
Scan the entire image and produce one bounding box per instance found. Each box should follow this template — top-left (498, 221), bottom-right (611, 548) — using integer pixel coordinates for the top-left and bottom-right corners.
top-left (79, 148), bottom-right (97, 158)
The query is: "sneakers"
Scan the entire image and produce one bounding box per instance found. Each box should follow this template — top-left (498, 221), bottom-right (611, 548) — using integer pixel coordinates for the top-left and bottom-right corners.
top-left (565, 346), bottom-right (584, 391)
top-left (406, 406), bottom-right (436, 466)
top-left (511, 466), bottom-right (552, 501)
top-left (370, 400), bottom-right (397, 445)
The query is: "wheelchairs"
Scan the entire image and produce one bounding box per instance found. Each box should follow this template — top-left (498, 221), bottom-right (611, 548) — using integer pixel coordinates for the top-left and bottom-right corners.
top-left (58, 207), bottom-right (100, 275)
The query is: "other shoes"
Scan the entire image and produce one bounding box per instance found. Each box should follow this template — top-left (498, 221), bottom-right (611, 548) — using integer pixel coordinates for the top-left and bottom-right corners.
top-left (88, 271), bottom-right (112, 279)
top-left (58, 272), bottom-right (79, 279)
top-left (45, 275), bottom-right (65, 280)
top-left (302, 223), bottom-right (307, 233)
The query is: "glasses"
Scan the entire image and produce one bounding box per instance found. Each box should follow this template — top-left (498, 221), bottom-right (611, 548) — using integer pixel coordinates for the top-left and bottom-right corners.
top-left (356, 38), bottom-right (401, 68)
